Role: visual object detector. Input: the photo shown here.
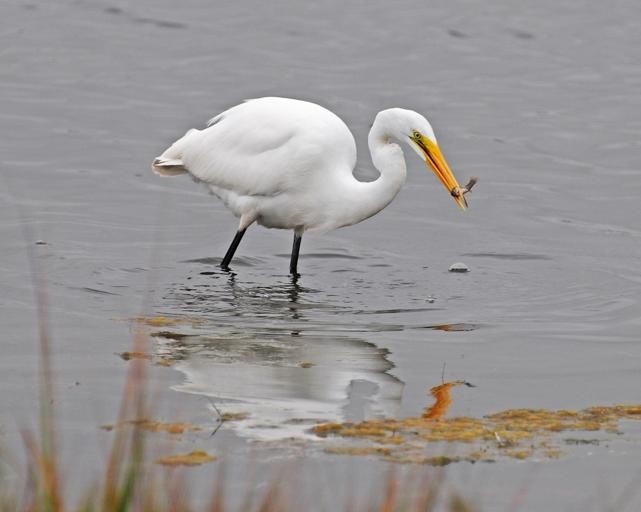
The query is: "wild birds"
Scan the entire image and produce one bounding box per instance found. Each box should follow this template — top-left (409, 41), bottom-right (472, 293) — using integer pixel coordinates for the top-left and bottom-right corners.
top-left (152, 96), bottom-right (469, 287)
